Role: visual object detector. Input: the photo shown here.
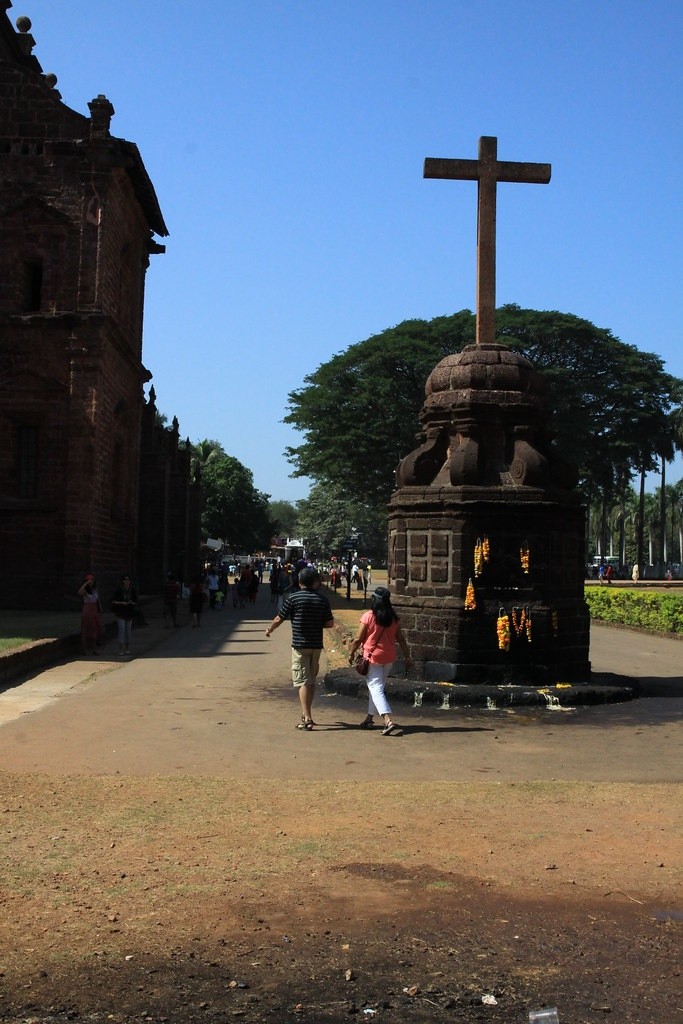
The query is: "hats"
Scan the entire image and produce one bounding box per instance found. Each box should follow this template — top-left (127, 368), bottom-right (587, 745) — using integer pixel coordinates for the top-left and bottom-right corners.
top-left (85, 575), bottom-right (94, 581)
top-left (370, 587), bottom-right (392, 600)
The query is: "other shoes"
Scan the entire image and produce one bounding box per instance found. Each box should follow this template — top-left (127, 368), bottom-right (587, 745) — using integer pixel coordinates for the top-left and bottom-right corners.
top-left (92, 650), bottom-right (101, 656)
top-left (83, 651), bottom-right (90, 656)
top-left (164, 625), bottom-right (170, 629)
top-left (118, 651), bottom-right (123, 655)
top-left (125, 650), bottom-right (131, 654)
top-left (174, 624), bottom-right (181, 628)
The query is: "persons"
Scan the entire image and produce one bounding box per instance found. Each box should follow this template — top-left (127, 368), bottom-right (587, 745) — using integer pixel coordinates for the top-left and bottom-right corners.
top-left (77, 554), bottom-right (372, 655)
top-left (349, 587), bottom-right (414, 736)
top-left (598, 561), bottom-right (672, 586)
top-left (266, 568), bottom-right (334, 731)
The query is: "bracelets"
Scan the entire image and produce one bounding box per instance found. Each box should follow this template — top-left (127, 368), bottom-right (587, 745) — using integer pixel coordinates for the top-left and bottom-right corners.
top-left (406, 656), bottom-right (410, 657)
top-left (349, 653), bottom-right (354, 655)
top-left (267, 627), bottom-right (271, 632)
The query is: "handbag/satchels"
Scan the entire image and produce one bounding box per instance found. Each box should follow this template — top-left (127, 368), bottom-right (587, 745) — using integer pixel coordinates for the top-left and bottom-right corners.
top-left (354, 652), bottom-right (370, 675)
top-left (200, 588), bottom-right (209, 603)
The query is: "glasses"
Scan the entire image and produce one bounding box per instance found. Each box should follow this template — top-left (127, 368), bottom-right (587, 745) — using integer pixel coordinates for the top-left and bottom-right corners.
top-left (124, 578), bottom-right (130, 581)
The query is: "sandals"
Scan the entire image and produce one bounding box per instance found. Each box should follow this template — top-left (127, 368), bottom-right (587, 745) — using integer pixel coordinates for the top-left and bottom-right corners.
top-left (360, 721), bottom-right (376, 730)
top-left (381, 720), bottom-right (398, 736)
top-left (302, 715), bottom-right (313, 724)
top-left (295, 720), bottom-right (314, 731)
